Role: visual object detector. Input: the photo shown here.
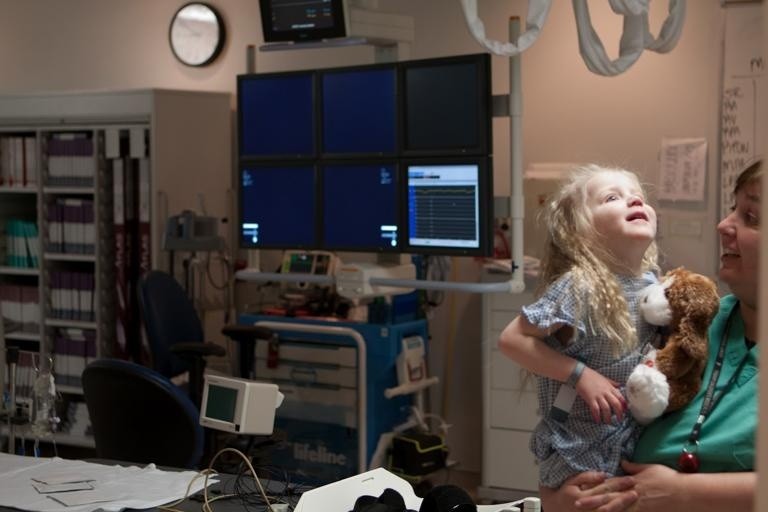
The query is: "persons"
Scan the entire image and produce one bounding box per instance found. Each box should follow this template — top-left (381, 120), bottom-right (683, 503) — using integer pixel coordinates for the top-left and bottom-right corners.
top-left (497, 163), bottom-right (666, 512)
top-left (574, 164), bottom-right (767, 512)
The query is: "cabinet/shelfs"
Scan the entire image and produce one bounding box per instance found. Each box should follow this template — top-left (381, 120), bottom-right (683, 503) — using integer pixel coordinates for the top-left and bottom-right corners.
top-left (251, 338), bottom-right (361, 488)
top-left (0, 88), bottom-right (234, 455)
top-left (476, 272), bottom-right (542, 505)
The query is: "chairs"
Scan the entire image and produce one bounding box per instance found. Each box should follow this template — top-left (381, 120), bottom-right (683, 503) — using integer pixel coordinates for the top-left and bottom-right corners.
top-left (80, 359), bottom-right (206, 470)
top-left (136, 269), bottom-right (229, 412)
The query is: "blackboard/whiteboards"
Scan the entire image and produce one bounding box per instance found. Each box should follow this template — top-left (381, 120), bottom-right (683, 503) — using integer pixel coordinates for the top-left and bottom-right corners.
top-left (713, 0), bottom-right (768, 297)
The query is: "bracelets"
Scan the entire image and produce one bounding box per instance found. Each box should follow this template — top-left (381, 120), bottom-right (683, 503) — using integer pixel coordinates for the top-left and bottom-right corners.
top-left (567, 361), bottom-right (585, 387)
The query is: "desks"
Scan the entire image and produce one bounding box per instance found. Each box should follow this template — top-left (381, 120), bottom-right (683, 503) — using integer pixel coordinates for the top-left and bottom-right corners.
top-left (0, 457), bottom-right (319, 512)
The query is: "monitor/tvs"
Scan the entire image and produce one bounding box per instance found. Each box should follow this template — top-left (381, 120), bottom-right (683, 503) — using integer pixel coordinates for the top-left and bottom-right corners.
top-left (199, 375), bottom-right (285, 435)
top-left (231, 52), bottom-right (494, 257)
top-left (259, 0), bottom-right (346, 42)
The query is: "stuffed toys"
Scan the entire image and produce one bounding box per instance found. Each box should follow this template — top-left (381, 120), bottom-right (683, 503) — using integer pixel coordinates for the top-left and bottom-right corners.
top-left (626, 266), bottom-right (720, 424)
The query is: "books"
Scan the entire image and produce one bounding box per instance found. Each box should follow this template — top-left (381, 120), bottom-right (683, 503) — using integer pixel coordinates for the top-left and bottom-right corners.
top-left (0, 133), bottom-right (96, 438)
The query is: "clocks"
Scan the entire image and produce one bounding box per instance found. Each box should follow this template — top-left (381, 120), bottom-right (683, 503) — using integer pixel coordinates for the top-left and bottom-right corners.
top-left (169, 2), bottom-right (226, 67)
